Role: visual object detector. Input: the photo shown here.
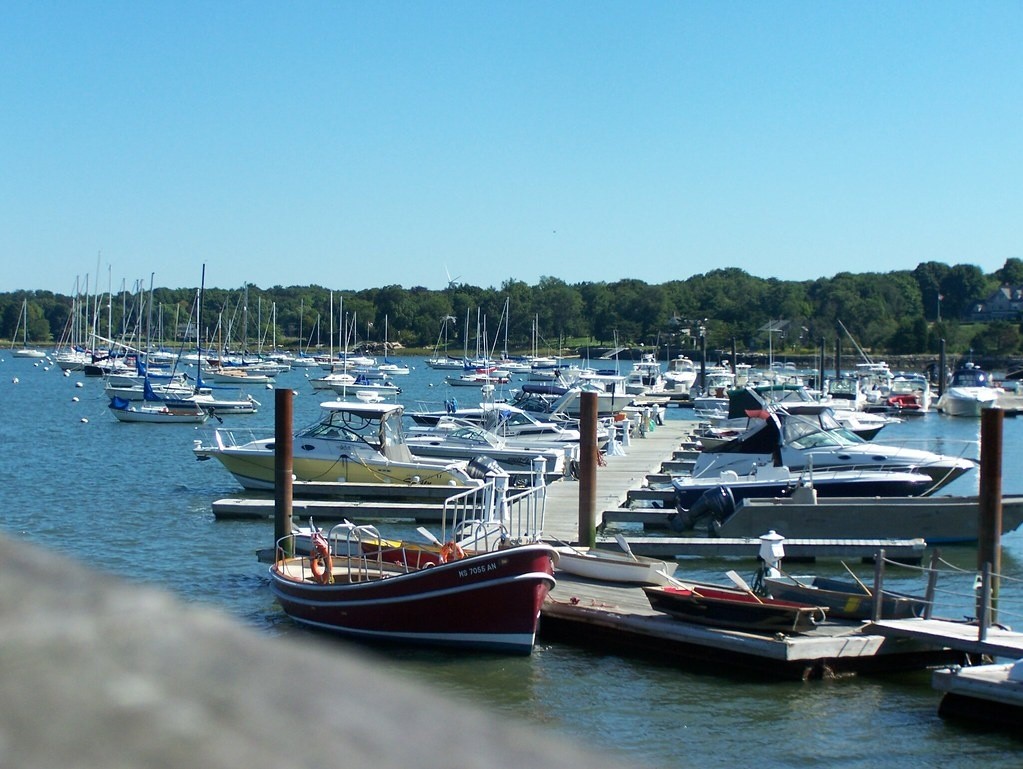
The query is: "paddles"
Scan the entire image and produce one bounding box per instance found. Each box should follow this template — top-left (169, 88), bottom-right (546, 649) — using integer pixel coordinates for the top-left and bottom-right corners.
top-left (614, 533), bottom-right (638, 563)
top-left (840, 560), bottom-right (871, 596)
top-left (360, 528), bottom-right (396, 547)
top-left (655, 569), bottom-right (704, 597)
top-left (343, 519), bottom-right (359, 542)
top-left (725, 569), bottom-right (763, 604)
top-left (549, 534), bottom-right (585, 557)
top-left (291, 523), bottom-right (302, 533)
top-left (416, 526), bottom-right (443, 548)
top-left (767, 561), bottom-right (807, 587)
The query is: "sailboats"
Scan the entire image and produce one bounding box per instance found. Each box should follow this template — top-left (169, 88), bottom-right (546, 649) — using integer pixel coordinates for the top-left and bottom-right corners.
top-left (49, 248), bottom-right (1023, 508)
top-left (7, 296), bottom-right (47, 358)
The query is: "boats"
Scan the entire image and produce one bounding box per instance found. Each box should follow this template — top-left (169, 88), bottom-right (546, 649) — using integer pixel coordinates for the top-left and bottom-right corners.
top-left (642, 579), bottom-right (832, 635)
top-left (190, 311), bottom-right (519, 503)
top-left (266, 514), bottom-right (560, 656)
top-left (763, 573), bottom-right (926, 623)
top-left (663, 451), bottom-right (1022, 547)
top-left (291, 523), bottom-right (486, 570)
top-left (552, 544), bottom-right (680, 586)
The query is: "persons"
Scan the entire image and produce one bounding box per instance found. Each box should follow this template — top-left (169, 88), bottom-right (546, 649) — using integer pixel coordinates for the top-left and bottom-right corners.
top-left (808, 376), bottom-right (814, 387)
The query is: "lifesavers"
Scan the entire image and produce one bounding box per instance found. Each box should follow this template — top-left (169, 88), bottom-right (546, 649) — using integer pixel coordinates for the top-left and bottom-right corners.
top-left (309, 544), bottom-right (331, 585)
top-left (439, 541), bottom-right (464, 564)
